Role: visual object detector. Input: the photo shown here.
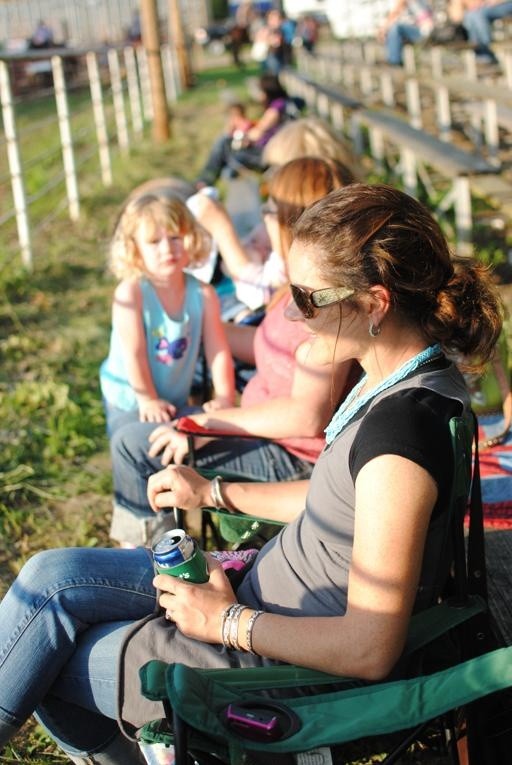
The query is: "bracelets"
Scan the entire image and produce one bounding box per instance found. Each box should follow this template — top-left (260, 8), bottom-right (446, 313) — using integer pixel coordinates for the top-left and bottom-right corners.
top-left (220, 602), bottom-right (266, 658)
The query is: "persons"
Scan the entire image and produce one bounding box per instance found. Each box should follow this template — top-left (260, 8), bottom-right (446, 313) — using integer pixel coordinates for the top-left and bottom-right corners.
top-left (197, 1), bottom-right (321, 75)
top-left (0, 183), bottom-right (474, 764)
top-left (122, 7), bottom-right (141, 43)
top-left (194, 69), bottom-right (306, 191)
top-left (98, 119), bottom-right (368, 548)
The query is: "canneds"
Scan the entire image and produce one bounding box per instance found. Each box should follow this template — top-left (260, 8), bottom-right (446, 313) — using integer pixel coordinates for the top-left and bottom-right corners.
top-left (150, 527), bottom-right (193, 571)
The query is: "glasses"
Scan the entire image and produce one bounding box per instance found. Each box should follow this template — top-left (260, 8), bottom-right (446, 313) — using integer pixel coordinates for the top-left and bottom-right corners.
top-left (290, 283), bottom-right (356, 320)
